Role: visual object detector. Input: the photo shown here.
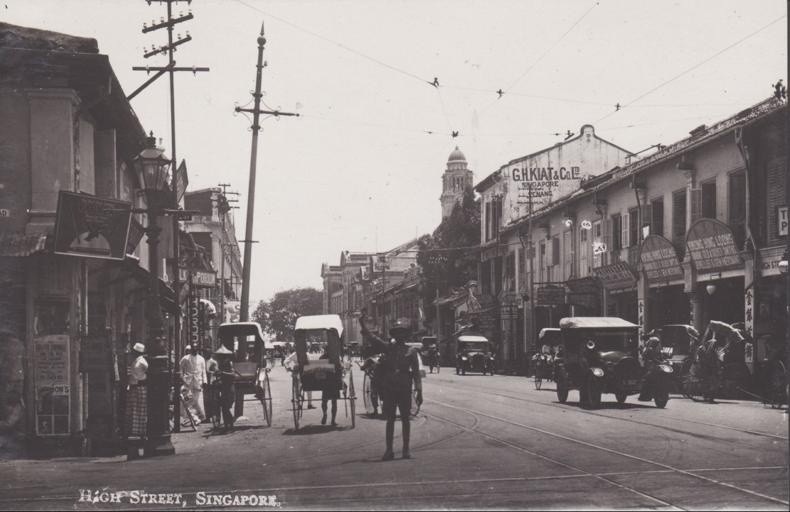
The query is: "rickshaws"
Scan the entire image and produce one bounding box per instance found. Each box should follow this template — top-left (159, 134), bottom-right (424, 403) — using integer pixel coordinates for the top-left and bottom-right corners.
top-left (530, 324), bottom-right (566, 391)
top-left (640, 317), bottom-right (789, 411)
top-left (354, 351), bottom-right (432, 421)
top-left (285, 312), bottom-right (359, 432)
top-left (198, 322), bottom-right (274, 430)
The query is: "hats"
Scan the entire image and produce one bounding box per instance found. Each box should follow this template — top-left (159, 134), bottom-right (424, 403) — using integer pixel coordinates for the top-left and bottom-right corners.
top-left (213, 344), bottom-right (233, 354)
top-left (133, 343), bottom-right (145, 353)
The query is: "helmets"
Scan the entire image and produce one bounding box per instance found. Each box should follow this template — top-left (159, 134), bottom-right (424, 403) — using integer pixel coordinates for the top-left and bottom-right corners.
top-left (389, 317), bottom-right (412, 335)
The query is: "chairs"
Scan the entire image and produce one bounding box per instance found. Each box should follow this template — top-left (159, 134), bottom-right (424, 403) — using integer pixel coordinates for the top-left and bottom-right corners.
top-left (234, 362), bottom-right (258, 380)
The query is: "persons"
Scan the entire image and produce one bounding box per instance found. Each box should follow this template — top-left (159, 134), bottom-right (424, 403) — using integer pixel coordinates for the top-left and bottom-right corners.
top-left (358, 314), bottom-right (422, 459)
top-left (285, 343), bottom-right (317, 409)
top-left (126, 341), bottom-right (150, 435)
top-left (180, 342), bottom-right (208, 423)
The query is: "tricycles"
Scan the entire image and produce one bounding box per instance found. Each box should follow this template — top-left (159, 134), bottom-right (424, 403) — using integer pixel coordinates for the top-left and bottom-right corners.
top-left (419, 336), bottom-right (442, 374)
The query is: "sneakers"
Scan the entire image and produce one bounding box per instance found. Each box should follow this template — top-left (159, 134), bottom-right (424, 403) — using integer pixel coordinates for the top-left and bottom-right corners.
top-left (321, 415), bottom-right (327, 424)
top-left (308, 405), bottom-right (316, 408)
top-left (402, 450), bottom-right (414, 458)
top-left (383, 452), bottom-right (394, 460)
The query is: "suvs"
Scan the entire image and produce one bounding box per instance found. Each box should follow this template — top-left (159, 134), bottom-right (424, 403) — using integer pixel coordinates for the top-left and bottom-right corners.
top-left (554, 316), bottom-right (671, 413)
top-left (452, 331), bottom-right (498, 376)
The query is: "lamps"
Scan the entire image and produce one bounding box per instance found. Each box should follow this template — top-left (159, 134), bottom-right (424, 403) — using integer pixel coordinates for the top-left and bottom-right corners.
top-left (706, 271), bottom-right (722, 295)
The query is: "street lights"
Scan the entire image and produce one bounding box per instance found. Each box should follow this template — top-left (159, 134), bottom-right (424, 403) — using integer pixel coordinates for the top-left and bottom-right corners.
top-left (129, 127), bottom-right (192, 463)
